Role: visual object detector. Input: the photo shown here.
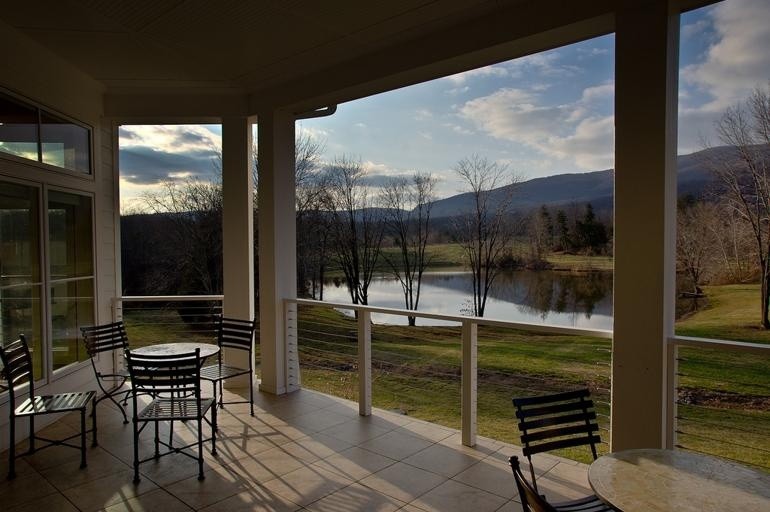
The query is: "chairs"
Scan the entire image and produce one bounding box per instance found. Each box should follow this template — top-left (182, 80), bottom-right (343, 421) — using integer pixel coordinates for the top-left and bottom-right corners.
top-left (0, 320), bottom-right (217, 486)
top-left (192, 313), bottom-right (257, 417)
top-left (508, 389), bottom-right (614, 512)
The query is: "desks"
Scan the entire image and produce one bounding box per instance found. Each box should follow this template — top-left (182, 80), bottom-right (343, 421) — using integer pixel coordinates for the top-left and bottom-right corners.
top-left (588, 448), bottom-right (769, 512)
top-left (124, 341), bottom-right (221, 452)
